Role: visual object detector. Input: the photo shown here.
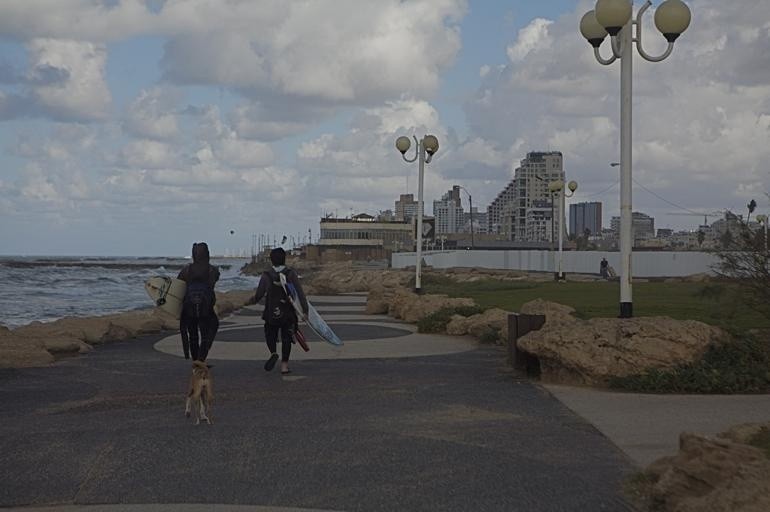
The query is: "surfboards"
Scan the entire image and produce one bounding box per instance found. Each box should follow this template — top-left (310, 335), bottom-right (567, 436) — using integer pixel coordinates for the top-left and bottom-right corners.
top-left (280, 273), bottom-right (344, 346)
top-left (145, 275), bottom-right (219, 320)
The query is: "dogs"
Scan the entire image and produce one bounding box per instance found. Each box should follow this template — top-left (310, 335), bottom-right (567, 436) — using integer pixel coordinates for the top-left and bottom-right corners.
top-left (185, 360), bottom-right (216, 426)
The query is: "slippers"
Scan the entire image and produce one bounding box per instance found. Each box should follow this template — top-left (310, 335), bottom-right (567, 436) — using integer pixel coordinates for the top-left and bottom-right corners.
top-left (280, 365), bottom-right (292, 373)
top-left (264, 353), bottom-right (279, 371)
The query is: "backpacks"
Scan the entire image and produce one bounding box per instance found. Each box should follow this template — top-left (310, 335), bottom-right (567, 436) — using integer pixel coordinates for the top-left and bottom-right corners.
top-left (264, 269), bottom-right (291, 321)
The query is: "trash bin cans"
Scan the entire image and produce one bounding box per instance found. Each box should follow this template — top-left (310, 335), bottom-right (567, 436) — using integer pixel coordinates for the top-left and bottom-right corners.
top-left (509, 315), bottom-right (546, 378)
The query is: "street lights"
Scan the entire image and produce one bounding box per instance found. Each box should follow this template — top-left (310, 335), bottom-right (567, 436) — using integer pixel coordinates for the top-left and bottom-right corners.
top-left (548, 178), bottom-right (578, 282)
top-left (455, 186), bottom-right (474, 250)
top-left (395, 130), bottom-right (439, 292)
top-left (580, 1), bottom-right (690, 320)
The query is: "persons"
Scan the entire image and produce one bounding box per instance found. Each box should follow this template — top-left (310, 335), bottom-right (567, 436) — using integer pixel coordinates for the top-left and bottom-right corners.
top-left (246, 248), bottom-right (309, 375)
top-left (175, 241), bottom-right (221, 370)
top-left (600, 258), bottom-right (608, 279)
